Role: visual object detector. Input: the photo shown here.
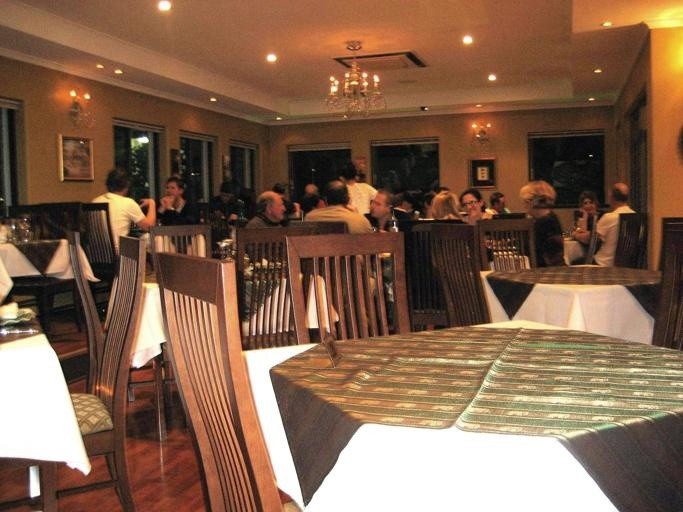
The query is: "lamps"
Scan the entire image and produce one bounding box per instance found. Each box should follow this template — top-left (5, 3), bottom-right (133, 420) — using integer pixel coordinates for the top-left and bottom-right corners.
top-left (326, 41), bottom-right (387, 119)
top-left (333, 52), bottom-right (426, 73)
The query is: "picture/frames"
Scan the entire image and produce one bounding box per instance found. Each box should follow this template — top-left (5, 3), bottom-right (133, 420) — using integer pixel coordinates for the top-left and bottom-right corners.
top-left (58, 134), bottom-right (94, 182)
top-left (467, 156), bottom-right (497, 190)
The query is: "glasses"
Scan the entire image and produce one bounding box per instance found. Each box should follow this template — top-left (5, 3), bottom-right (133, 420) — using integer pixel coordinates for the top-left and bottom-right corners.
top-left (462, 201), bottom-right (480, 209)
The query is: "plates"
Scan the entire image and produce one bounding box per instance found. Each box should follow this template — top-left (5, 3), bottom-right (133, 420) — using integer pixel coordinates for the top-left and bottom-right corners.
top-left (0, 307), bottom-right (38, 327)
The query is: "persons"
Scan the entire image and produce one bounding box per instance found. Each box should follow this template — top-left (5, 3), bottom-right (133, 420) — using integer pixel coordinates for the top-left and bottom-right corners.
top-left (89, 169), bottom-right (158, 264)
top-left (206, 160), bottom-right (511, 340)
top-left (568, 183), bottom-right (643, 268)
top-left (157, 176), bottom-right (199, 226)
top-left (519, 180), bottom-right (567, 267)
top-left (574, 190), bottom-right (605, 231)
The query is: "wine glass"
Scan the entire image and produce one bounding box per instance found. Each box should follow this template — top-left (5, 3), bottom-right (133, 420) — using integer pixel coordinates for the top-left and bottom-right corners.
top-left (216, 239), bottom-right (235, 259)
top-left (5, 214), bottom-right (33, 245)
